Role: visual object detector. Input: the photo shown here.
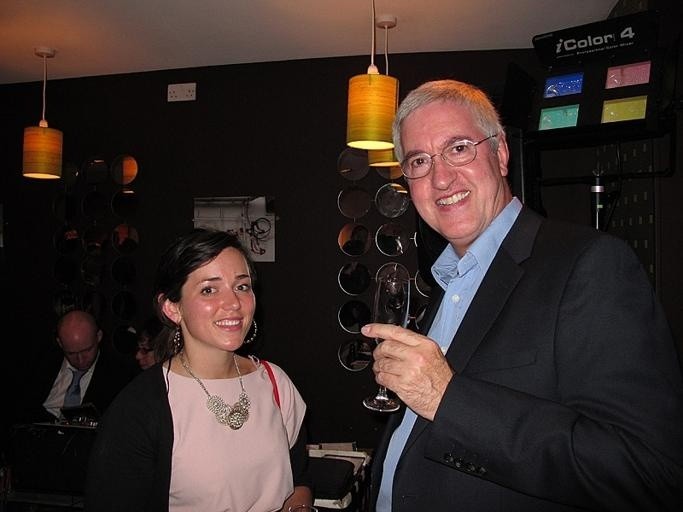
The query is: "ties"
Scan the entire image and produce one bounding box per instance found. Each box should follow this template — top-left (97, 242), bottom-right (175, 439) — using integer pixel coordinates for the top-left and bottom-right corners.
top-left (60, 370), bottom-right (88, 420)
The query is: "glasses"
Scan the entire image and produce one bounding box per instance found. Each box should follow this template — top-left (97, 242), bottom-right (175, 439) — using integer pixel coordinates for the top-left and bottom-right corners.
top-left (399, 134), bottom-right (499, 179)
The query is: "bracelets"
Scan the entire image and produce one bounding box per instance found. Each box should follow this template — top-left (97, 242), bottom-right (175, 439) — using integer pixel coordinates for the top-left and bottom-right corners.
top-left (288, 504), bottom-right (319, 512)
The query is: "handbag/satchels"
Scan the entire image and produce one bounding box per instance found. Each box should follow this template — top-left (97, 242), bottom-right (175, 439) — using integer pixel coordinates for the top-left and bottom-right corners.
top-left (297, 456), bottom-right (355, 498)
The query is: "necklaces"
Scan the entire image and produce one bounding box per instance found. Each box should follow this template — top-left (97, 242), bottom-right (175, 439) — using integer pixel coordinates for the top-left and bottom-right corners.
top-left (178, 350), bottom-right (251, 429)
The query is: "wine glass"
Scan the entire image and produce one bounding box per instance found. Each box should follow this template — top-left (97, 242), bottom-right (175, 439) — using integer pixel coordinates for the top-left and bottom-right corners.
top-left (362, 275), bottom-right (410, 413)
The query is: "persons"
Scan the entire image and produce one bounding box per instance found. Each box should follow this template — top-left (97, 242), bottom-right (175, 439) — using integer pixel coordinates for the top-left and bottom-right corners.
top-left (79, 226), bottom-right (320, 512)
top-left (361, 76), bottom-right (682, 507)
top-left (31, 310), bottom-right (123, 436)
top-left (133, 324), bottom-right (165, 370)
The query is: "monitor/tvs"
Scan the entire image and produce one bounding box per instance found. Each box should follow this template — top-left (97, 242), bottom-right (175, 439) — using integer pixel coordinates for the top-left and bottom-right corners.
top-left (605, 59), bottom-right (652, 91)
top-left (600, 94), bottom-right (650, 126)
top-left (537, 103), bottom-right (580, 133)
top-left (543, 72), bottom-right (585, 98)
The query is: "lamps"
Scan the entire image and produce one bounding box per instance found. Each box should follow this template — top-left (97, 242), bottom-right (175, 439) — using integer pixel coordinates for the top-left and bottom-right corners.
top-left (345, 0), bottom-right (405, 168)
top-left (22, 43), bottom-right (65, 182)
